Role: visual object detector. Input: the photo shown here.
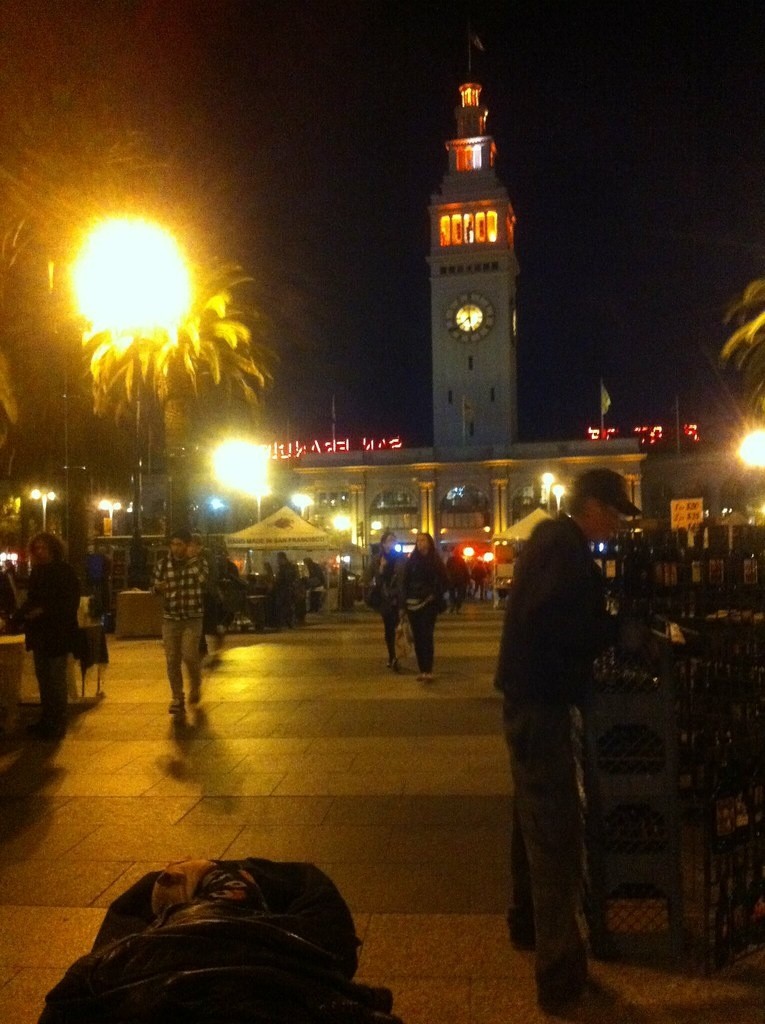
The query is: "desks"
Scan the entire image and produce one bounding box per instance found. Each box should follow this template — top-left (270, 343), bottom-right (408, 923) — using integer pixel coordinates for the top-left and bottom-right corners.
top-left (249, 593), bottom-right (267, 631)
top-left (113, 590), bottom-right (163, 639)
top-left (71, 624), bottom-right (109, 697)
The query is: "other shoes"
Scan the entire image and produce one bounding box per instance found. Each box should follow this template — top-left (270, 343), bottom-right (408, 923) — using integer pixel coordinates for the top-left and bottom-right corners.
top-left (542, 977), bottom-right (602, 1015)
top-left (416, 671), bottom-right (433, 682)
top-left (386, 657), bottom-right (400, 670)
top-left (168, 692), bottom-right (185, 712)
top-left (188, 685), bottom-right (200, 703)
top-left (507, 925), bottom-right (538, 949)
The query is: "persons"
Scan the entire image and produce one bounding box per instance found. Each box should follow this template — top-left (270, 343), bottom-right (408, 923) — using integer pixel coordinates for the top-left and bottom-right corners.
top-left (446, 546), bottom-right (473, 615)
top-left (218, 551), bottom-right (241, 631)
top-left (150, 529), bottom-right (210, 713)
top-left (86, 540), bottom-right (110, 621)
top-left (473, 560), bottom-right (488, 602)
top-left (402, 532), bottom-right (445, 680)
top-left (0, 560), bottom-right (19, 627)
top-left (275, 549), bottom-right (300, 629)
top-left (358, 532), bottom-right (407, 669)
top-left (303, 557), bottom-right (326, 614)
top-left (493, 467), bottom-right (644, 1013)
top-left (0, 530), bottom-right (83, 742)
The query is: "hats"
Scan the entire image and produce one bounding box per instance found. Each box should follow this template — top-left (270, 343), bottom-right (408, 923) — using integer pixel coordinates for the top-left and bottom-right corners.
top-left (576, 467), bottom-right (641, 515)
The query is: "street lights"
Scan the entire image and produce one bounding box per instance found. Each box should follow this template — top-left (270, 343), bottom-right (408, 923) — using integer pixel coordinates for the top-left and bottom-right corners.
top-left (31, 489), bottom-right (55, 532)
top-left (102, 501), bottom-right (121, 536)
top-left (294, 492), bottom-right (313, 519)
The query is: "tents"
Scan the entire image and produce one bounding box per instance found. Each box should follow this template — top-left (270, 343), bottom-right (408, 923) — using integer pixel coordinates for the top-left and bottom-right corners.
top-left (493, 507), bottom-right (557, 607)
top-left (224, 505), bottom-right (343, 616)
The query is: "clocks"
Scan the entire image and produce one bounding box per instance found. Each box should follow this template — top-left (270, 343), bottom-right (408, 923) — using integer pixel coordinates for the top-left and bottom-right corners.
top-left (444, 293), bottom-right (496, 346)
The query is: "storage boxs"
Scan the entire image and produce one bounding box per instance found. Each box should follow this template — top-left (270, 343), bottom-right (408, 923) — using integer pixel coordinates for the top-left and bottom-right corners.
top-left (587, 690), bottom-right (683, 962)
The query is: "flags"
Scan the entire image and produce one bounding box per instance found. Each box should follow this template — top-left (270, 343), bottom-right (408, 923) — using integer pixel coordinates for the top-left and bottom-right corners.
top-left (333, 401), bottom-right (336, 440)
top-left (601, 384), bottom-right (611, 418)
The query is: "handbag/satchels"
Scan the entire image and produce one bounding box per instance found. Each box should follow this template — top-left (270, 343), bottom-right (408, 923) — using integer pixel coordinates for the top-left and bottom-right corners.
top-left (437, 595), bottom-right (447, 613)
top-left (395, 614), bottom-right (415, 657)
top-left (406, 595), bottom-right (433, 611)
top-left (76, 595), bottom-right (101, 628)
top-left (365, 577), bottom-right (390, 608)
